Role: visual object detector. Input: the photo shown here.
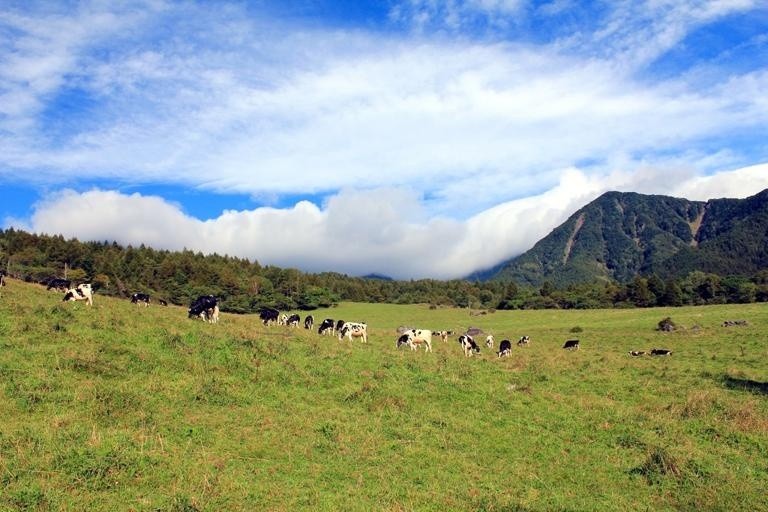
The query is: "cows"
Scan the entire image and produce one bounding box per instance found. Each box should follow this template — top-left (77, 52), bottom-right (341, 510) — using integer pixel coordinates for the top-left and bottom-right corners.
top-left (650, 348), bottom-right (673, 357)
top-left (562, 340), bottom-right (579, 351)
top-left (628, 351), bottom-right (646, 358)
top-left (395, 327), bottom-right (532, 359)
top-left (187, 294), bottom-right (221, 324)
top-left (259, 305), bottom-right (314, 331)
top-left (318, 317), bottom-right (367, 345)
top-left (46, 278), bottom-right (71, 292)
top-left (158, 299), bottom-right (167, 306)
top-left (128, 293), bottom-right (150, 308)
top-left (62, 283), bottom-right (94, 306)
